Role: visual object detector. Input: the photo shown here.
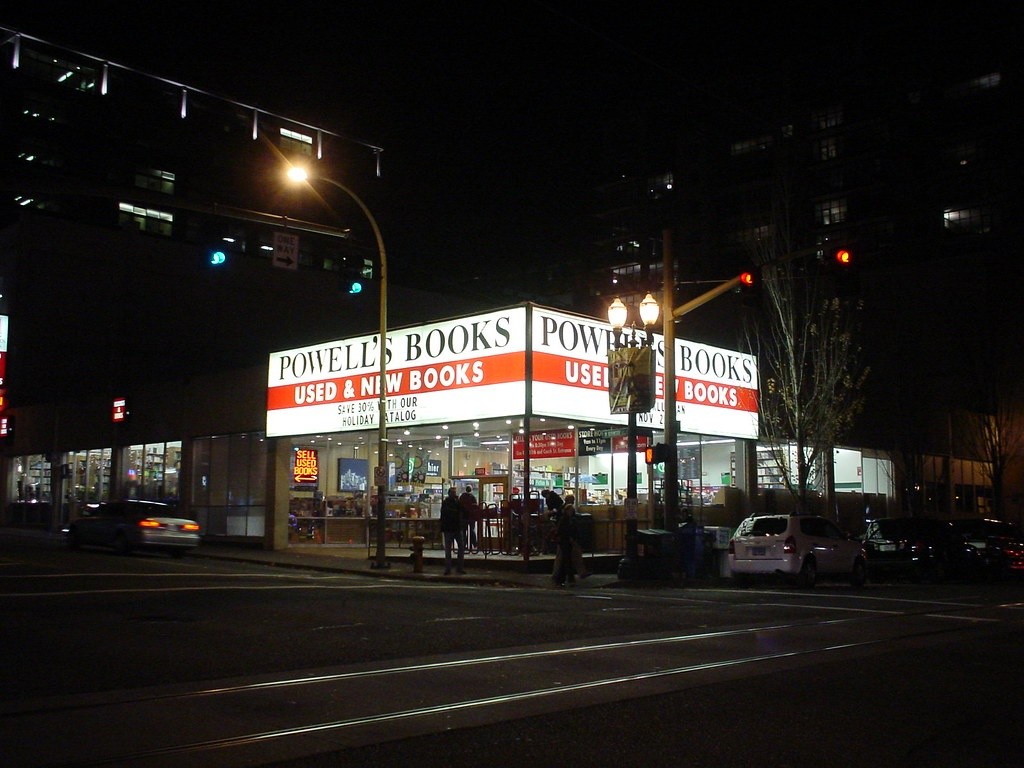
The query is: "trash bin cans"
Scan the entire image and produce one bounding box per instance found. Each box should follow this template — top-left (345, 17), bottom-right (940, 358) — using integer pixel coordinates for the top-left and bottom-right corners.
top-left (637, 526), bottom-right (733, 580)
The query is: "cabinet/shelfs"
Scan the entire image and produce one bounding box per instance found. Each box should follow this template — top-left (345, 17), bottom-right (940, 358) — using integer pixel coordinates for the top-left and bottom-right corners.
top-left (490, 469), bottom-right (582, 510)
top-left (290, 496), bottom-right (446, 549)
top-left (15, 447), bottom-right (181, 502)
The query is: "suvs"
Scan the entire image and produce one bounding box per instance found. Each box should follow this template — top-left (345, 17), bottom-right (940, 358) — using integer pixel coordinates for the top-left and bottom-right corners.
top-left (727, 512), bottom-right (869, 590)
top-left (861, 518), bottom-right (987, 585)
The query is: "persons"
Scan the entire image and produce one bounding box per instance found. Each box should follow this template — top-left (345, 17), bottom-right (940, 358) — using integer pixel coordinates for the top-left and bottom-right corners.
top-left (460, 486), bottom-right (477, 548)
top-left (552, 504), bottom-right (580, 582)
top-left (295, 497), bottom-right (378, 518)
top-left (542, 490), bottom-right (564, 510)
top-left (556, 495), bottom-right (575, 558)
top-left (439, 487), bottom-right (466, 574)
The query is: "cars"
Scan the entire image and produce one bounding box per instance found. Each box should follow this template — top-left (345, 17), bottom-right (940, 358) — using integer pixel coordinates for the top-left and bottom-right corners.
top-left (62, 499), bottom-right (199, 557)
top-left (948, 519), bottom-right (1024, 583)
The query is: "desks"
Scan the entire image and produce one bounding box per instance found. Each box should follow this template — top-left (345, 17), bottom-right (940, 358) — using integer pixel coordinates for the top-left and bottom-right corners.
top-left (600, 519), bottom-right (652, 554)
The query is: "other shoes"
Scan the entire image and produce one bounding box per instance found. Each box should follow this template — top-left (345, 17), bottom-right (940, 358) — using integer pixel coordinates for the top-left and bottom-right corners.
top-left (457, 568), bottom-right (466, 574)
top-left (444, 569), bottom-right (451, 576)
top-left (580, 572), bottom-right (592, 579)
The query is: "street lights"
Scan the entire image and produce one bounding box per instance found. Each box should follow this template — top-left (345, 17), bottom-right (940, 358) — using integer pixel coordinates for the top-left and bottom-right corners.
top-left (275, 154), bottom-right (387, 569)
top-left (605, 293), bottom-right (661, 576)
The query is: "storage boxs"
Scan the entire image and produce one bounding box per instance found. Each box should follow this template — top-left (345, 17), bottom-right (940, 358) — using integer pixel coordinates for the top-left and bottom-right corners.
top-left (431, 504), bottom-right (442, 518)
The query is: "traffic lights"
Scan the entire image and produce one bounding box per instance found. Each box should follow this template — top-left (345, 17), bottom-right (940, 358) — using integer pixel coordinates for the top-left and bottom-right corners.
top-left (341, 261), bottom-right (367, 295)
top-left (646, 446), bottom-right (661, 464)
top-left (207, 238), bottom-right (229, 275)
top-left (838, 245), bottom-right (854, 269)
top-left (739, 261), bottom-right (760, 293)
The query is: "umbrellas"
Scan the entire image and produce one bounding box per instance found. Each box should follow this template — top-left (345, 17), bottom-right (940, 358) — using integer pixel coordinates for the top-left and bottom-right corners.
top-left (570, 474), bottom-right (601, 489)
